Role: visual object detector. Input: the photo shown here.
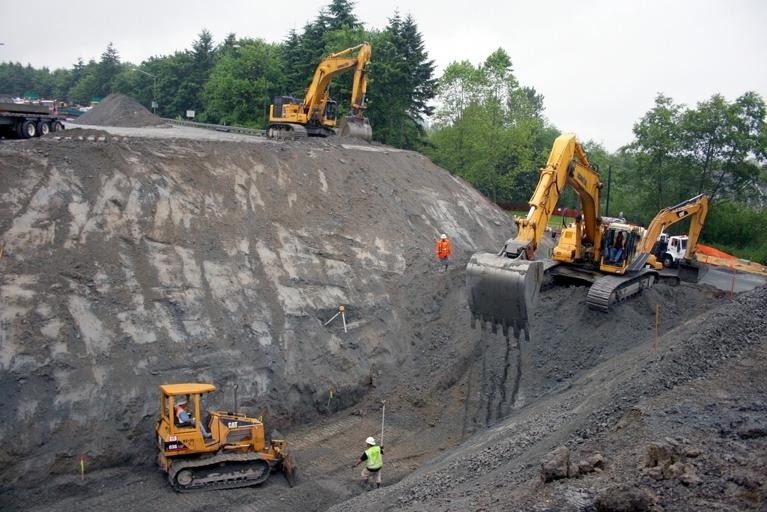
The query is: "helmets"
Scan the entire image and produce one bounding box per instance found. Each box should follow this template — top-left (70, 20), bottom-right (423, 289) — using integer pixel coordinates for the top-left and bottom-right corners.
top-left (366, 437), bottom-right (375, 445)
top-left (441, 234), bottom-right (447, 239)
top-left (178, 399), bottom-right (187, 405)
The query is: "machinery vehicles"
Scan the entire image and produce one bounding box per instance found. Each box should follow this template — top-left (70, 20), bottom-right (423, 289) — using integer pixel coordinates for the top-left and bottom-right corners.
top-left (0, 102), bottom-right (64, 138)
top-left (266, 41), bottom-right (373, 141)
top-left (466, 135), bottom-right (708, 342)
top-left (155, 383), bottom-right (331, 492)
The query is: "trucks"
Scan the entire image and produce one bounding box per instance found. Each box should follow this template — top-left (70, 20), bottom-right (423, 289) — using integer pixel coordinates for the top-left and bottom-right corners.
top-left (609, 222), bottom-right (689, 268)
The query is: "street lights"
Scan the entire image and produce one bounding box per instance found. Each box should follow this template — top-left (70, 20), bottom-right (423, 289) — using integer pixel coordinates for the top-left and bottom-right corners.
top-left (131, 69), bottom-right (156, 114)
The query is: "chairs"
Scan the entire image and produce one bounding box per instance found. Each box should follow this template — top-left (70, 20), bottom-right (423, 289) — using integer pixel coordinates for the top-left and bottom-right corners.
top-left (172, 408), bottom-right (193, 429)
top-left (609, 231), bottom-right (631, 262)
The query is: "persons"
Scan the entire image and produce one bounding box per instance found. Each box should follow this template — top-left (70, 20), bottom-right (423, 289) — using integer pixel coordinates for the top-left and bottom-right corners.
top-left (435, 234), bottom-right (453, 271)
top-left (608, 231), bottom-right (627, 262)
top-left (353, 437), bottom-right (385, 489)
top-left (547, 231), bottom-right (558, 258)
top-left (175, 399), bottom-right (213, 438)
